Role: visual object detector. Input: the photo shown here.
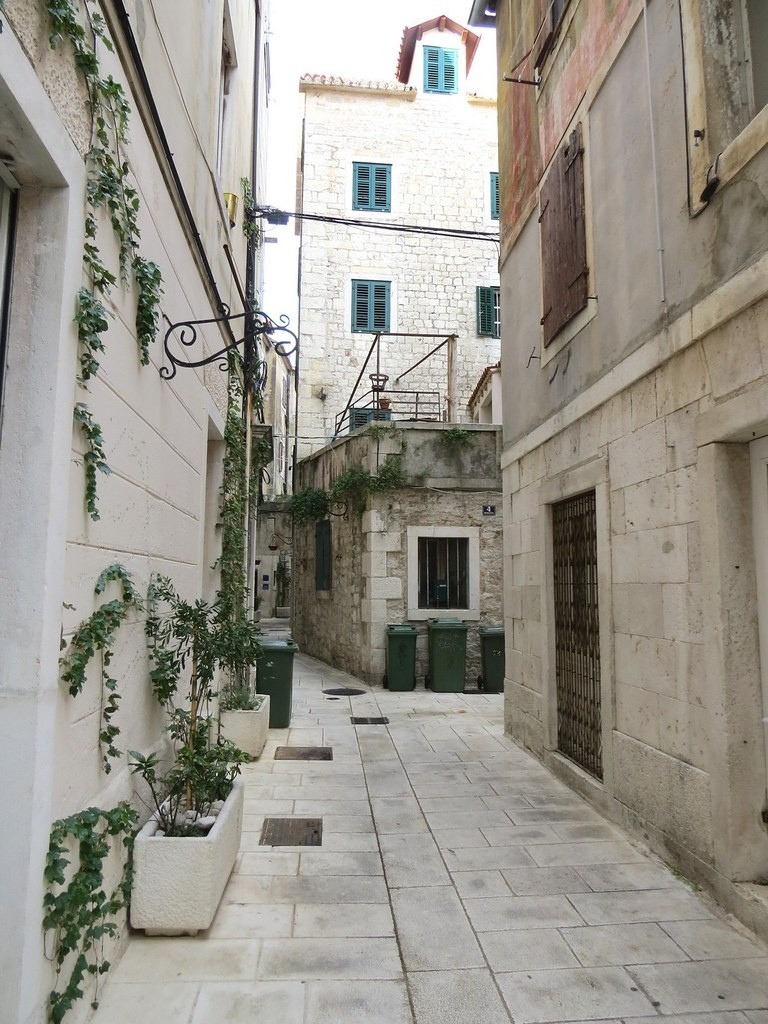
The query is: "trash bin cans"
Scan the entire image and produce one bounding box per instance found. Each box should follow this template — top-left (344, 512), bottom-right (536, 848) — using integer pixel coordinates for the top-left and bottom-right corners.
top-left (383, 623), bottom-right (419, 692)
top-left (475, 625), bottom-right (505, 692)
top-left (424, 617), bottom-right (471, 693)
top-left (255, 636), bottom-right (299, 730)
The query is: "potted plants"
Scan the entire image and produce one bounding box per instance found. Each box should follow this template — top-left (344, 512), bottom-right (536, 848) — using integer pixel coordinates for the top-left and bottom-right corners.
top-left (255, 595), bottom-right (264, 622)
top-left (128, 596), bottom-right (246, 938)
top-left (210, 590), bottom-right (271, 759)
